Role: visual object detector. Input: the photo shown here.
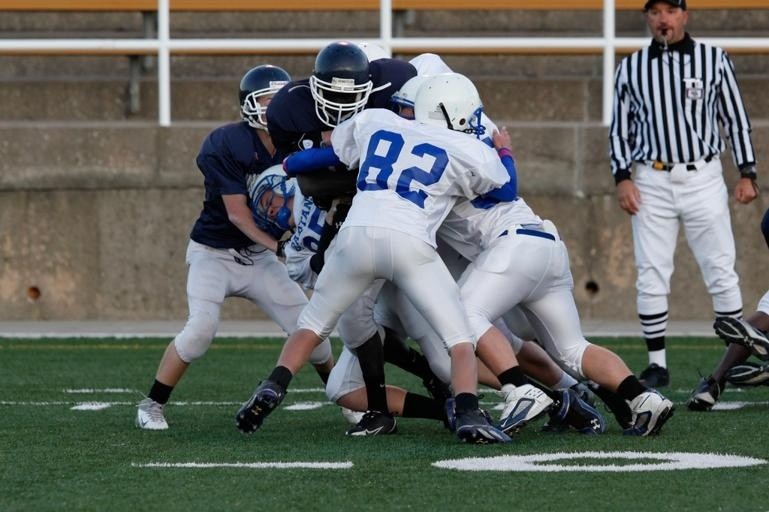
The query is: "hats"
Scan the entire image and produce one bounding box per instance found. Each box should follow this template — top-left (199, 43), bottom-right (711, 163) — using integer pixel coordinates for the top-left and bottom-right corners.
top-left (644, 0), bottom-right (687, 11)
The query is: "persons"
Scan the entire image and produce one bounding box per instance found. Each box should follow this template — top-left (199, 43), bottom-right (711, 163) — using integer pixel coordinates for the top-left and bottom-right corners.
top-left (235, 41), bottom-right (674, 444)
top-left (713, 316), bottom-right (769, 389)
top-left (609, 0), bottom-right (759, 389)
top-left (685, 209), bottom-right (769, 410)
top-left (135, 63), bottom-right (367, 431)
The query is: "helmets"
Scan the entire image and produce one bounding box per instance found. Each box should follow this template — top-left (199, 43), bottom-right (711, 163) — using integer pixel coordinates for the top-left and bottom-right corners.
top-left (389, 73), bottom-right (434, 121)
top-left (308, 39), bottom-right (374, 128)
top-left (238, 64), bottom-right (292, 130)
top-left (413, 73), bottom-right (487, 141)
top-left (247, 164), bottom-right (306, 240)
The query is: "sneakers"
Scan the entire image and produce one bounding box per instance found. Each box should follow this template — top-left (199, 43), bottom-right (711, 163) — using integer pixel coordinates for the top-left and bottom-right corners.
top-left (233, 378), bottom-right (288, 436)
top-left (683, 376), bottom-right (723, 412)
top-left (133, 395), bottom-right (169, 432)
top-left (454, 418), bottom-right (512, 447)
top-left (713, 315), bottom-right (769, 363)
top-left (496, 382), bottom-right (556, 438)
top-left (601, 383), bottom-right (676, 438)
top-left (723, 362), bottom-right (769, 388)
top-left (543, 382), bottom-right (606, 435)
top-left (636, 361), bottom-right (670, 388)
top-left (442, 395), bottom-right (492, 433)
top-left (341, 404), bottom-right (398, 438)
top-left (418, 354), bottom-right (453, 400)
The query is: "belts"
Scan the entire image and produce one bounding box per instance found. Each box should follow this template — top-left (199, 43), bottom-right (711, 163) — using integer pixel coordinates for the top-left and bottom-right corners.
top-left (636, 154), bottom-right (718, 171)
